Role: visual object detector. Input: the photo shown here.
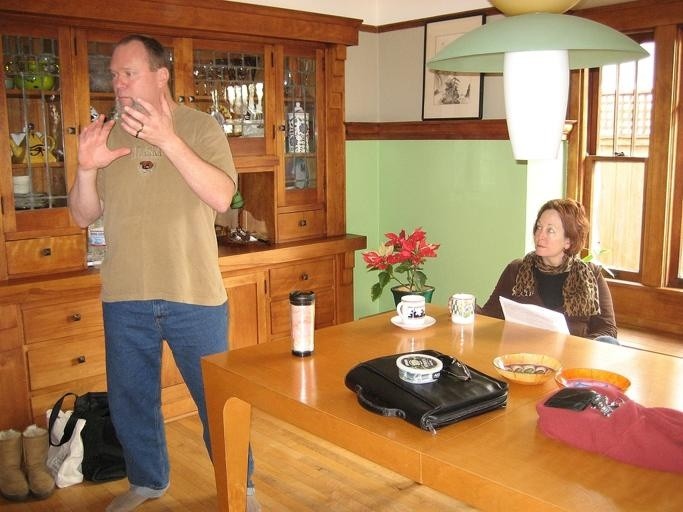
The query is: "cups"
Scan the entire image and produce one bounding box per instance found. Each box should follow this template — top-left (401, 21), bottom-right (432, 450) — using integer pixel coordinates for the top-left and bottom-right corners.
top-left (397, 294), bottom-right (427, 324)
top-left (289, 290), bottom-right (316, 358)
top-left (12, 176), bottom-right (31, 193)
top-left (449, 294), bottom-right (476, 324)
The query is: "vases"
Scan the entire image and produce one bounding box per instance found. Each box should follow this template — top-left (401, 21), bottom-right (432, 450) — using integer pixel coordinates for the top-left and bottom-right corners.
top-left (391, 284), bottom-right (436, 309)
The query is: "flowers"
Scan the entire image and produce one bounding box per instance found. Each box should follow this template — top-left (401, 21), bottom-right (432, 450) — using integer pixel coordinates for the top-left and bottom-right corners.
top-left (361, 225), bottom-right (440, 302)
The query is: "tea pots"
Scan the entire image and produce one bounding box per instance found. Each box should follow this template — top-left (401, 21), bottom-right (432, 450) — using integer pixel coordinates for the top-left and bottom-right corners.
top-left (9, 123), bottom-right (58, 163)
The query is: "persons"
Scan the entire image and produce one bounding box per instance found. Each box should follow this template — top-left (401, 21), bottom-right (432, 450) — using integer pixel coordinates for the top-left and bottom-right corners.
top-left (65, 34), bottom-right (262, 512)
top-left (475, 197), bottom-right (620, 345)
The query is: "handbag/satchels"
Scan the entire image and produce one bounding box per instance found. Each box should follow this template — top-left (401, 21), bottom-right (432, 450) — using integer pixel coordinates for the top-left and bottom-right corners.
top-left (76, 392), bottom-right (127, 483)
top-left (46, 389), bottom-right (85, 490)
top-left (342, 347), bottom-right (511, 433)
top-left (533, 378), bottom-right (681, 470)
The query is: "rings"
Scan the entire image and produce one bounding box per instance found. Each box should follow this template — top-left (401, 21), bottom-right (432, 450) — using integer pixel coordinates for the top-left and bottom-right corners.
top-left (138, 123), bottom-right (143, 132)
top-left (136, 131), bottom-right (139, 138)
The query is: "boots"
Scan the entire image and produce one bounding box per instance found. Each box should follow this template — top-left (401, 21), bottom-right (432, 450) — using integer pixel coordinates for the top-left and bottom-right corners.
top-left (0, 429), bottom-right (30, 501)
top-left (22, 424), bottom-right (55, 500)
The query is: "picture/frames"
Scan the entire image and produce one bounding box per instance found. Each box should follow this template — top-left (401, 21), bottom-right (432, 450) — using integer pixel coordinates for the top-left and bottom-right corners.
top-left (422, 10), bottom-right (487, 121)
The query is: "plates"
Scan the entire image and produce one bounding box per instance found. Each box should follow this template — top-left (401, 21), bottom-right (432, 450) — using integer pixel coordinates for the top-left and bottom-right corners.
top-left (493, 353), bottom-right (561, 386)
top-left (554, 367), bottom-right (630, 394)
top-left (390, 314), bottom-right (436, 329)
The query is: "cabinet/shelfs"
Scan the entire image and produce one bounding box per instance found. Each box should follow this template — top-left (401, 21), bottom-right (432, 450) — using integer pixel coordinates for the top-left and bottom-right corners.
top-left (0, 16), bottom-right (88, 281)
top-left (240, 43), bottom-right (345, 246)
top-left (267, 249), bottom-right (354, 343)
top-left (74, 29), bottom-right (278, 176)
top-left (0, 305), bottom-right (33, 432)
top-left (22, 265), bottom-right (264, 428)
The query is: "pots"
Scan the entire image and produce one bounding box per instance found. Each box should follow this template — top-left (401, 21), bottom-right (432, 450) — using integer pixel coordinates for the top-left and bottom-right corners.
top-left (13, 72), bottom-right (57, 91)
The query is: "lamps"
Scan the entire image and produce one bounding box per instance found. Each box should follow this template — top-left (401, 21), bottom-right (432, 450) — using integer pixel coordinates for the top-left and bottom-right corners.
top-left (426, 1), bottom-right (651, 163)
